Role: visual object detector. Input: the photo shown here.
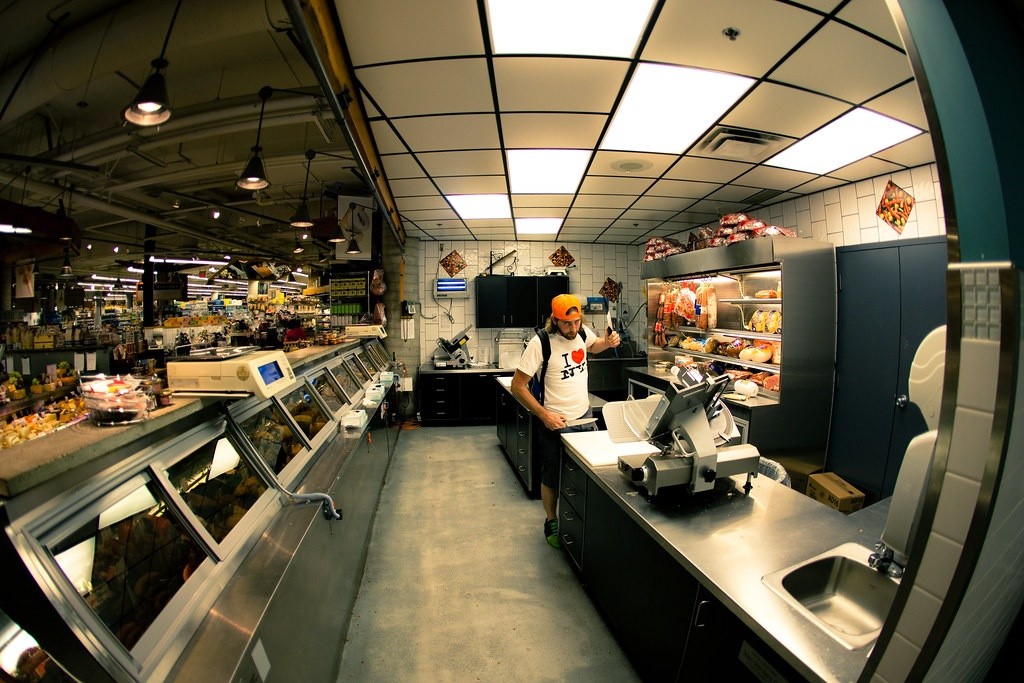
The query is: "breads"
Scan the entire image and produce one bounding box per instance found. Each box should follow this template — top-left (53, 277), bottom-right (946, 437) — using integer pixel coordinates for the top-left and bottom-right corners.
top-left (224, 406), bottom-right (329, 533)
top-left (644, 214), bottom-right (785, 260)
top-left (654, 286), bottom-right (782, 393)
top-left (0, 397), bottom-right (85, 449)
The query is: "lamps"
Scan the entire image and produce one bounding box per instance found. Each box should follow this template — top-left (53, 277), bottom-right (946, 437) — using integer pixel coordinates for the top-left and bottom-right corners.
top-left (326, 219), bottom-right (347, 243)
top-left (60, 252), bottom-right (74, 277)
top-left (290, 152), bottom-right (317, 228)
top-left (148, 255), bottom-right (231, 266)
top-left (346, 202), bottom-right (361, 254)
top-left (124, 0), bottom-right (182, 127)
top-left (235, 85), bottom-right (275, 191)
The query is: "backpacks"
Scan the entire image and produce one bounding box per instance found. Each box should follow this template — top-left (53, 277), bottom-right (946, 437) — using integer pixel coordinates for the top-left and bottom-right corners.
top-left (532, 325), bottom-right (587, 404)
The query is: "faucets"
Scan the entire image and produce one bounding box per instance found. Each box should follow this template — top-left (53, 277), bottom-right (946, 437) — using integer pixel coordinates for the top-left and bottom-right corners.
top-left (868, 540), bottom-right (895, 572)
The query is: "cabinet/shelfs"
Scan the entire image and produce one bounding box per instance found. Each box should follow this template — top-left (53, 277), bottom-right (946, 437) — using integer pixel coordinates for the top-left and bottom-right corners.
top-left (678, 583), bottom-right (811, 683)
top-left (419, 373), bottom-right (460, 427)
top-left (461, 372), bottom-right (515, 426)
top-left (517, 403), bottom-right (532, 500)
top-left (330, 270), bottom-right (371, 326)
top-left (558, 446), bottom-right (585, 592)
top-left (507, 394), bottom-right (517, 472)
top-left (496, 380), bottom-right (510, 458)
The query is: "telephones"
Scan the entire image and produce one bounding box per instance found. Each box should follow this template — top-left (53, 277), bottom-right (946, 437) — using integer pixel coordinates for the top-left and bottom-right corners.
top-left (402, 300), bottom-right (416, 316)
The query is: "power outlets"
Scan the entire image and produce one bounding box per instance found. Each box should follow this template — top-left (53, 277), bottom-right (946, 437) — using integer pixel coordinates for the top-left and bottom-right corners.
top-left (440, 244), bottom-right (445, 252)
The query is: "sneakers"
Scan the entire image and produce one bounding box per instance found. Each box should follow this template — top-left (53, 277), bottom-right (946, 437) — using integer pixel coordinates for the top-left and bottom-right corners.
top-left (544, 518), bottom-right (560, 549)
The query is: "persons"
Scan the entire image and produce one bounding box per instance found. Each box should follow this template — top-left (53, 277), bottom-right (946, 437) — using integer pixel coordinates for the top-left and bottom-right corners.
top-left (510, 294), bottom-right (621, 548)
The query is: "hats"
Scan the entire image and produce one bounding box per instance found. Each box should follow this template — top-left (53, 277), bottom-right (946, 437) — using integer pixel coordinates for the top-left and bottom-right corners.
top-left (551, 293), bottom-right (583, 321)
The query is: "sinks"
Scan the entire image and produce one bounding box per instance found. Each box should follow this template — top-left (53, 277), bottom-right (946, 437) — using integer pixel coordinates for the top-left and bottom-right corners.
top-left (782, 553), bottom-right (900, 638)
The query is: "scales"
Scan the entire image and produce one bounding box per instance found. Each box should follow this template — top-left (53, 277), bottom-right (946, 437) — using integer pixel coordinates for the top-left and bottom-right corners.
top-left (345, 324), bottom-right (388, 340)
top-left (166, 347), bottom-right (297, 402)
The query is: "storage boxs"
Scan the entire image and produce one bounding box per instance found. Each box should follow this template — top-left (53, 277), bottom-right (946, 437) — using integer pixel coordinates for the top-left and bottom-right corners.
top-left (806, 472), bottom-right (865, 512)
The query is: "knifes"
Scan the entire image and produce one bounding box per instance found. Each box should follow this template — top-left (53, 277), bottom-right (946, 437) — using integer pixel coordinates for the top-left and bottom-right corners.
top-left (563, 418), bottom-right (598, 426)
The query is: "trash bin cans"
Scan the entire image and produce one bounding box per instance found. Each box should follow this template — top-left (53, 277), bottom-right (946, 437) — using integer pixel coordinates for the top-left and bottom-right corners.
top-left (758, 456), bottom-right (786, 484)
